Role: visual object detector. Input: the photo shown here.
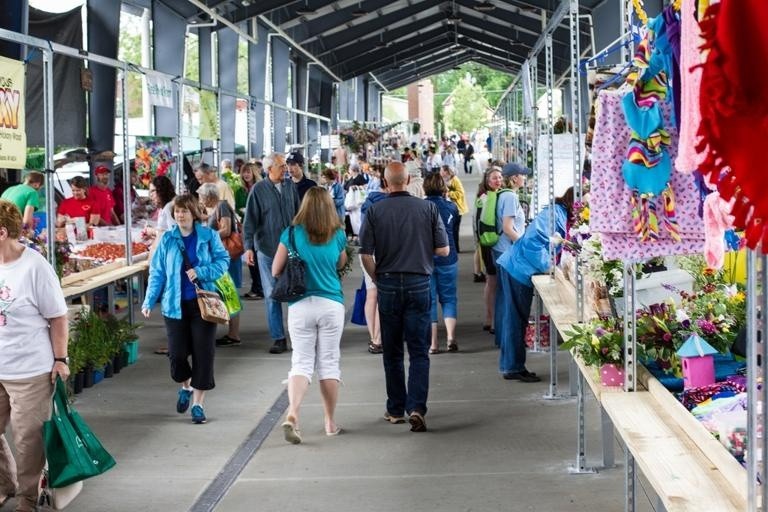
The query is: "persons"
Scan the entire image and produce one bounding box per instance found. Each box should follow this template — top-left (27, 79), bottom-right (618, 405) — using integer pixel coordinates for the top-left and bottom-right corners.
top-left (342, 163), bottom-right (367, 243)
top-left (0, 200), bottom-right (71, 511)
top-left (321, 168), bottom-right (345, 224)
top-left (486, 133), bottom-right (492, 160)
top-left (140, 193), bottom-right (231, 424)
top-left (286, 151), bottom-right (318, 203)
top-left (110, 165), bottom-right (137, 223)
top-left (479, 169), bottom-right (503, 331)
top-left (359, 172), bottom-right (389, 354)
top-left (481, 162), bottom-right (533, 335)
top-left (0, 170), bottom-right (44, 236)
top-left (421, 168), bottom-right (462, 354)
top-left (244, 152), bottom-right (300, 354)
top-left (222, 160), bottom-right (231, 170)
top-left (88, 165), bottom-right (120, 225)
top-left (402, 133), bottom-right (473, 167)
top-left (495, 187), bottom-right (574, 383)
top-left (235, 163), bottom-right (264, 299)
top-left (473, 160), bottom-right (503, 283)
top-left (271, 186), bottom-right (348, 445)
top-left (196, 183), bottom-right (241, 347)
top-left (329, 155), bottom-right (339, 181)
top-left (440, 164), bottom-right (469, 253)
top-left (401, 160), bottom-right (422, 200)
top-left (140, 175), bottom-right (178, 356)
top-left (56, 177), bottom-right (101, 227)
top-left (359, 164), bottom-right (450, 432)
top-left (193, 163), bottom-right (235, 214)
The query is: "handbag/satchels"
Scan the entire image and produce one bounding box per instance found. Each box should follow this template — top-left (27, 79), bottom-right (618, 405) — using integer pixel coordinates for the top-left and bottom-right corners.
top-left (175, 238), bottom-right (230, 326)
top-left (216, 200), bottom-right (245, 260)
top-left (41, 375), bottom-right (117, 489)
top-left (270, 224), bottom-right (307, 303)
top-left (35, 400), bottom-right (84, 510)
top-left (214, 270), bottom-right (243, 319)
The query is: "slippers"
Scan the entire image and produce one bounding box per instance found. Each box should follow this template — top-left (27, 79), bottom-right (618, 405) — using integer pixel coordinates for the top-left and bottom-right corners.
top-left (489, 328), bottom-right (495, 334)
top-left (281, 421), bottom-right (302, 445)
top-left (325, 424), bottom-right (342, 437)
top-left (242, 291), bottom-right (263, 300)
top-left (483, 325), bottom-right (492, 331)
top-left (215, 335), bottom-right (242, 347)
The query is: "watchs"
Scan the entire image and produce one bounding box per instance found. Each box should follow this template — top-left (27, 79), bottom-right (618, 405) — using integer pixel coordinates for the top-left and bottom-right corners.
top-left (54, 357), bottom-right (70, 364)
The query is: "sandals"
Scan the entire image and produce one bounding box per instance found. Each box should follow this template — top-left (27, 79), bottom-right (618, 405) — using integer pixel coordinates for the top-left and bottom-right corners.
top-left (371, 344), bottom-right (383, 354)
top-left (368, 340), bottom-right (373, 353)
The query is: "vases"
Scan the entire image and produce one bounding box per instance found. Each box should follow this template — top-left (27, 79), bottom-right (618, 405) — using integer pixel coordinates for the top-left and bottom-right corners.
top-left (596, 363), bottom-right (626, 386)
top-left (651, 348), bottom-right (669, 372)
top-left (606, 283), bottom-right (618, 320)
top-left (590, 295), bottom-right (611, 318)
top-left (668, 353), bottom-right (683, 380)
top-left (614, 297), bottom-right (629, 320)
top-left (349, 153), bottom-right (362, 171)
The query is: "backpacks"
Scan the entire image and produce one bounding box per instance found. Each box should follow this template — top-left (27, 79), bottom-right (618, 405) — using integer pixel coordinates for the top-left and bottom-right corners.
top-left (477, 189), bottom-right (519, 247)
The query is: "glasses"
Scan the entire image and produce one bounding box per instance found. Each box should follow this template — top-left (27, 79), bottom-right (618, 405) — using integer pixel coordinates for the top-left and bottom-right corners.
top-left (148, 189), bottom-right (157, 194)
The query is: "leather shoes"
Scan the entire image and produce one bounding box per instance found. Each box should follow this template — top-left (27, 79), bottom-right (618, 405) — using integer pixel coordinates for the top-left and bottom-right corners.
top-left (503, 368), bottom-right (541, 383)
top-left (503, 370), bottom-right (537, 380)
top-left (428, 343), bottom-right (440, 354)
top-left (447, 340), bottom-right (458, 353)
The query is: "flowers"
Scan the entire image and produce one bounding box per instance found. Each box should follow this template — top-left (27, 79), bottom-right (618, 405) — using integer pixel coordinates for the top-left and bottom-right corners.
top-left (580, 236), bottom-right (612, 290)
top-left (664, 321), bottom-right (730, 364)
top-left (328, 117), bottom-right (379, 155)
top-left (598, 250), bottom-right (664, 290)
top-left (664, 231), bottom-right (751, 342)
top-left (548, 197), bottom-right (598, 257)
top-left (561, 314), bottom-right (626, 365)
top-left (630, 299), bottom-right (679, 354)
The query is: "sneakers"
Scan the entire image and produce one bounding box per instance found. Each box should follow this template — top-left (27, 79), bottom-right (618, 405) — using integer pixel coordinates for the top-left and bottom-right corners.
top-left (472, 272), bottom-right (487, 283)
top-left (408, 408), bottom-right (427, 433)
top-left (190, 404), bottom-right (207, 425)
top-left (269, 338), bottom-right (287, 354)
top-left (176, 387), bottom-right (194, 413)
top-left (383, 411), bottom-right (407, 424)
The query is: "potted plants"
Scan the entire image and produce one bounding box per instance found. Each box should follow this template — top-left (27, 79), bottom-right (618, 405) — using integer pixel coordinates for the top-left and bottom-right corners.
top-left (69, 303), bottom-right (109, 386)
top-left (104, 314), bottom-right (125, 375)
top-left (60, 336), bottom-right (79, 397)
top-left (92, 314), bottom-right (115, 379)
top-left (68, 337), bottom-right (89, 396)
top-left (110, 316), bottom-right (143, 370)
top-left (66, 304), bottom-right (96, 394)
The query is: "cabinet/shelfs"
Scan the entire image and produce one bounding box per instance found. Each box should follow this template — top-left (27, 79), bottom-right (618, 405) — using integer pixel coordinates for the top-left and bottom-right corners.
top-left (524, 264), bottom-right (766, 512)
top-left (23, 230), bottom-right (155, 351)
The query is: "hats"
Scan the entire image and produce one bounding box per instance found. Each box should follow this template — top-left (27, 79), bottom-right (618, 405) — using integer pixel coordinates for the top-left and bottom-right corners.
top-left (285, 152), bottom-right (305, 165)
top-left (349, 163), bottom-right (359, 173)
top-left (501, 163), bottom-right (532, 177)
top-left (94, 165), bottom-right (112, 175)
top-left (321, 169), bottom-right (337, 180)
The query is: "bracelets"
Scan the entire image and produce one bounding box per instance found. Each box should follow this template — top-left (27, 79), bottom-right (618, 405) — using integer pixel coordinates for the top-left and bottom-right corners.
top-left (88, 222), bottom-right (96, 228)
